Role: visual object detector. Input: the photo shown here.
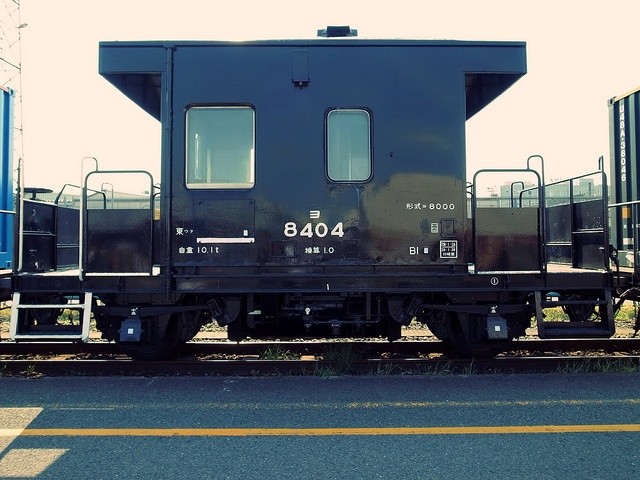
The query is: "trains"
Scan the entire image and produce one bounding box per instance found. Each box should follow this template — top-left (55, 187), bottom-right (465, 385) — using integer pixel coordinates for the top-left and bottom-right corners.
top-left (0, 26), bottom-right (640, 358)
